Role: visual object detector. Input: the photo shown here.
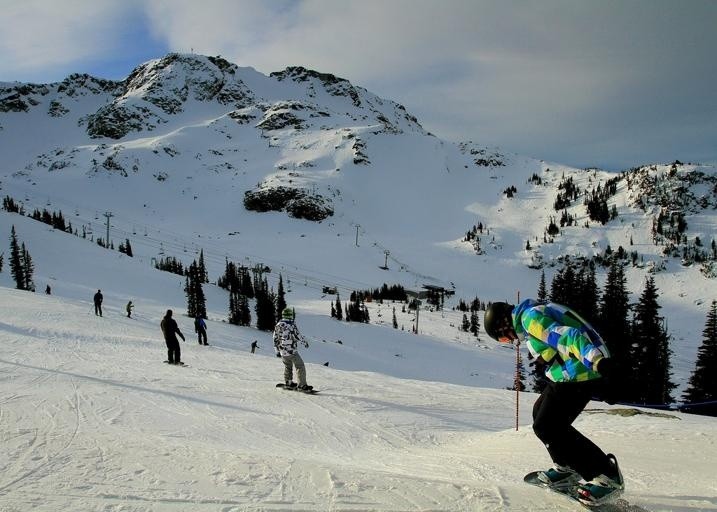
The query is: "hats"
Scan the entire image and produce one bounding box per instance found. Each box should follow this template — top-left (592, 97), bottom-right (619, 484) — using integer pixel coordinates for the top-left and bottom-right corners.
top-left (282, 307), bottom-right (293, 319)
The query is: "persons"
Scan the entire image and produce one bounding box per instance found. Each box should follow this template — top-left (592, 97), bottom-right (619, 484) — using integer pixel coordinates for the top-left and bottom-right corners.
top-left (480, 298), bottom-right (621, 504)
top-left (92, 288), bottom-right (104, 317)
top-left (124, 300), bottom-right (134, 318)
top-left (194, 313), bottom-right (209, 346)
top-left (249, 339), bottom-right (259, 354)
top-left (43, 283), bottom-right (52, 296)
top-left (272, 306), bottom-right (314, 391)
top-left (159, 308), bottom-right (186, 365)
top-left (27, 281), bottom-right (37, 293)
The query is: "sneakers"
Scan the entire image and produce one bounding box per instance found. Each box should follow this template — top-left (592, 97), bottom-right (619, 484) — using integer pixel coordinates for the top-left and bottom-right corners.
top-left (537, 468), bottom-right (618, 498)
top-left (298, 384), bottom-right (313, 390)
top-left (286, 380), bottom-right (297, 387)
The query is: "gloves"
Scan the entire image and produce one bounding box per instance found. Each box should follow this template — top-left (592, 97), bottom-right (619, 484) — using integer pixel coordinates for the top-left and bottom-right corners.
top-left (277, 352), bottom-right (281, 357)
top-left (305, 341), bottom-right (309, 348)
top-left (597, 358), bottom-right (629, 380)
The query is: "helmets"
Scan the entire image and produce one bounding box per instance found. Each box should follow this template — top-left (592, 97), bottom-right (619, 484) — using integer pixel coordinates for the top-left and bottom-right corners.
top-left (484, 302), bottom-right (519, 342)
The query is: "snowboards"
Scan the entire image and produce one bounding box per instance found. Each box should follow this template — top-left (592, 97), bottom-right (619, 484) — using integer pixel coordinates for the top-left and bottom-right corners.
top-left (523, 470), bottom-right (648, 512)
top-left (164, 360), bottom-right (189, 368)
top-left (276, 383), bottom-right (321, 393)
